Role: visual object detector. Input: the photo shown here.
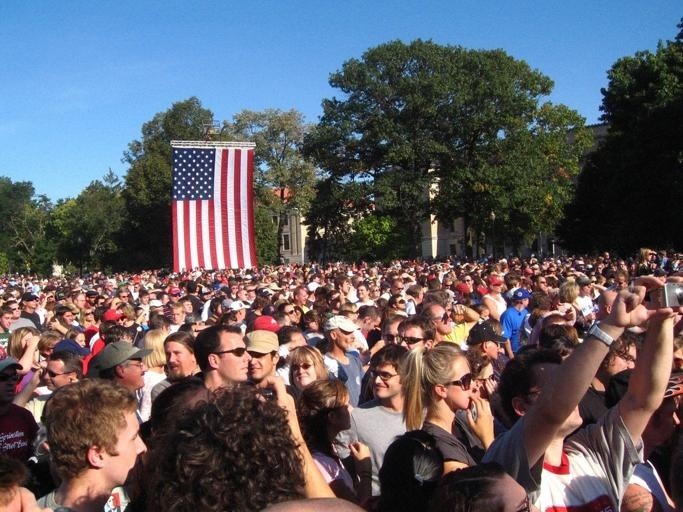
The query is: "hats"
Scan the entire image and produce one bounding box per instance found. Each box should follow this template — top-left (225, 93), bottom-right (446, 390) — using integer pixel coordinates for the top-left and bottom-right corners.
top-left (22, 292), bottom-right (39, 301)
top-left (103, 310), bottom-right (127, 321)
top-left (253, 315), bottom-right (280, 332)
top-left (269, 283), bottom-right (282, 291)
top-left (308, 282), bottom-right (321, 291)
top-left (56, 291), bottom-right (67, 301)
top-left (513, 288), bottom-right (533, 301)
top-left (222, 298), bottom-right (251, 311)
top-left (95, 341), bottom-right (154, 370)
top-left (457, 275), bottom-right (502, 293)
top-left (86, 291), bottom-right (100, 297)
top-left (245, 330), bottom-right (279, 354)
top-left (467, 324), bottom-right (507, 344)
top-left (604, 368), bottom-right (683, 407)
top-left (150, 287), bottom-right (180, 307)
top-left (325, 316), bottom-right (361, 332)
top-left (576, 275), bottom-right (594, 285)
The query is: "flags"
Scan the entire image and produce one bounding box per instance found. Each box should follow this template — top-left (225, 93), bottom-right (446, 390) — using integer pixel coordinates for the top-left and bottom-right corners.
top-left (170, 146), bottom-right (258, 273)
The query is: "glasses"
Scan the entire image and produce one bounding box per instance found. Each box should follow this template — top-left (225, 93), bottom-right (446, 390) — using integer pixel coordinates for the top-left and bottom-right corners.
top-left (217, 348), bottom-right (246, 357)
top-left (368, 369), bottom-right (398, 381)
top-left (45, 368), bottom-right (69, 378)
top-left (293, 363), bottom-right (310, 371)
top-left (432, 314), bottom-right (448, 324)
top-left (0, 373), bottom-right (18, 382)
top-left (518, 494), bottom-right (531, 512)
top-left (444, 373), bottom-right (472, 390)
top-left (0, 356), bottom-right (24, 371)
top-left (478, 373), bottom-right (495, 382)
top-left (618, 351), bottom-right (635, 363)
top-left (289, 309), bottom-right (297, 314)
top-left (386, 333), bottom-right (422, 344)
top-left (12, 307), bottom-right (20, 311)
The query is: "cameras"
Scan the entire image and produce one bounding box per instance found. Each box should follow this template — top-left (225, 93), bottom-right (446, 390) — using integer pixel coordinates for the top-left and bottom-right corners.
top-left (650, 283), bottom-right (683, 308)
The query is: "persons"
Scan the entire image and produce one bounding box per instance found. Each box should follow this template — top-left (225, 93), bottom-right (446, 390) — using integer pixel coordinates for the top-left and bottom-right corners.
top-left (2, 247), bottom-right (682, 512)
